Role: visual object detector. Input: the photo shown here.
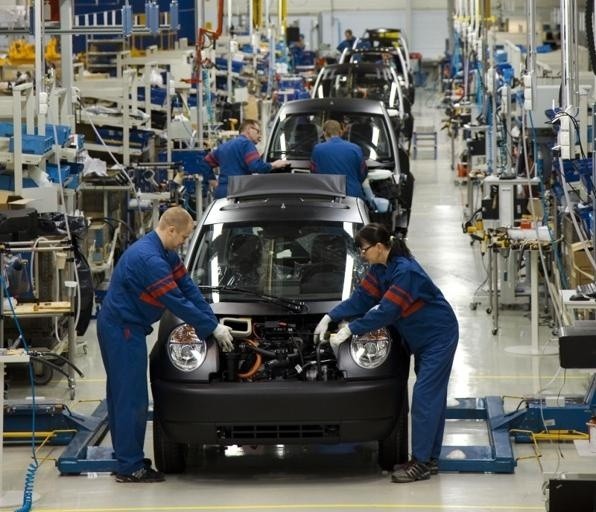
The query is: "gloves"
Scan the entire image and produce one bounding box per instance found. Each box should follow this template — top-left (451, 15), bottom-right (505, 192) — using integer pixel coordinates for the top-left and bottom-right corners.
top-left (213, 324), bottom-right (235, 352)
top-left (313, 314), bottom-right (331, 344)
top-left (332, 325), bottom-right (351, 347)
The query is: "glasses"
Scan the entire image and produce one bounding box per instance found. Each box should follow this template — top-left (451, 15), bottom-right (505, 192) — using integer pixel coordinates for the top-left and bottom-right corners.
top-left (359, 244), bottom-right (374, 255)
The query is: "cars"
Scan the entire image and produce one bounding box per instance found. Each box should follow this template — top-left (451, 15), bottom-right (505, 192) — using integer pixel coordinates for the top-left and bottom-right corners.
top-left (257, 95), bottom-right (415, 239)
top-left (147, 173), bottom-right (413, 476)
top-left (308, 27), bottom-right (416, 154)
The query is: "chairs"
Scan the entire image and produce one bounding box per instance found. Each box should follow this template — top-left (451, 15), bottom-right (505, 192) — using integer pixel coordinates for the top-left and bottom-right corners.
top-left (300, 233), bottom-right (346, 294)
top-left (348, 123), bottom-right (375, 156)
top-left (220, 235), bottom-right (268, 292)
top-left (291, 124), bottom-right (319, 155)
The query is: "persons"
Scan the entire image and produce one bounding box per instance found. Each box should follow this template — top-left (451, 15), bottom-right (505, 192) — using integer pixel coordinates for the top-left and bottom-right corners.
top-left (285, 27), bottom-right (396, 71)
top-left (94, 208), bottom-right (236, 484)
top-left (314, 223), bottom-right (459, 484)
top-left (308, 118), bottom-right (377, 208)
top-left (205, 118), bottom-right (291, 199)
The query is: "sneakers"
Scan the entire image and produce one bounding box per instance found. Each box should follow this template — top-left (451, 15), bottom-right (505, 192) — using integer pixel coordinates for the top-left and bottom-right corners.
top-left (111, 458), bottom-right (164, 481)
top-left (392, 456), bottom-right (438, 483)
